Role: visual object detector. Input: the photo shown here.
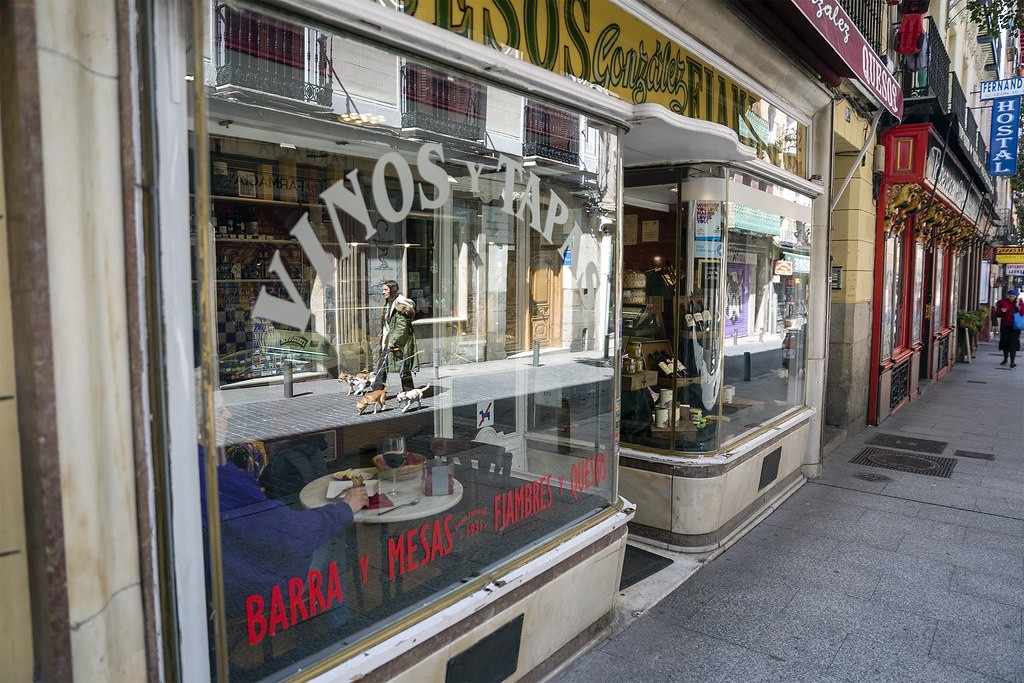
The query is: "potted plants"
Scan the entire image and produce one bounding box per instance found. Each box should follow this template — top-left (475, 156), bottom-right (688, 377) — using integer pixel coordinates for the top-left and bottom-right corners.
top-left (957, 305), bottom-right (989, 358)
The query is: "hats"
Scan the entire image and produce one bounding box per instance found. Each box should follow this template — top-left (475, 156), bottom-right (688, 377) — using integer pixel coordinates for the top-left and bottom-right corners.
top-left (1007, 290), bottom-right (1020, 296)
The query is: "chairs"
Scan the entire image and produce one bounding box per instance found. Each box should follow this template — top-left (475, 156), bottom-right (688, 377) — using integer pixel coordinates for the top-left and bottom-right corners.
top-left (394, 435), bottom-right (513, 585)
top-left (204, 527), bottom-right (346, 683)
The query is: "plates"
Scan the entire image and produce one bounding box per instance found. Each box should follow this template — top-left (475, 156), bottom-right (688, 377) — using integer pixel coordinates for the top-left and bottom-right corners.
top-left (327, 480), bottom-right (381, 499)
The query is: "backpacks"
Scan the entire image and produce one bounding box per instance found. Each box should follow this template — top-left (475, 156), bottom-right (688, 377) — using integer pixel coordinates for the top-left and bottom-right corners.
top-left (258, 441), bottom-right (329, 500)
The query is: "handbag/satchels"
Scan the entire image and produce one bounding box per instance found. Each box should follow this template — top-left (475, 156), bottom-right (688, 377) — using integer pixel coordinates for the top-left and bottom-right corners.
top-left (1012, 305), bottom-right (1024, 330)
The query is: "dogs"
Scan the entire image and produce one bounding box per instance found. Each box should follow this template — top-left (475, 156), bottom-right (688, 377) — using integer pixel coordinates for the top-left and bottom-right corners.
top-left (338, 370), bottom-right (369, 394)
top-left (356, 382), bottom-right (388, 415)
top-left (347, 372), bottom-right (376, 397)
top-left (395, 382), bottom-right (431, 413)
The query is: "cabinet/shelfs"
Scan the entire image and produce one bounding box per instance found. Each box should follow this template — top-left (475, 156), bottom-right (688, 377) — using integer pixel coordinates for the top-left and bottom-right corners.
top-left (188, 193), bottom-right (481, 383)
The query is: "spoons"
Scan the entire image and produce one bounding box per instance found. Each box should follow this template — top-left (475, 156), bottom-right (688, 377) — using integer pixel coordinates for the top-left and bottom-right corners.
top-left (377, 497), bottom-right (423, 517)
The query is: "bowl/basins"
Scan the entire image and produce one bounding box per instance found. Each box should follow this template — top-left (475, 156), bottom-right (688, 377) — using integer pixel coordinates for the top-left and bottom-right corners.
top-left (371, 452), bottom-right (426, 481)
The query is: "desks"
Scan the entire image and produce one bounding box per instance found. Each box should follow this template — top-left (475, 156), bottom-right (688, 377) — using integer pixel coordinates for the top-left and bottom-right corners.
top-left (300, 467), bottom-right (463, 616)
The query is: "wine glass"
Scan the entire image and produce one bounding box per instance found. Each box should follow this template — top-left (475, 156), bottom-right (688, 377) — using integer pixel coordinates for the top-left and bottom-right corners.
top-left (382, 434), bottom-right (407, 499)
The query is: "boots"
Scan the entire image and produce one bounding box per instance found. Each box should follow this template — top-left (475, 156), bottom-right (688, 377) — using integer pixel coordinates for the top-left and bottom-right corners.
top-left (1000, 356), bottom-right (1007, 365)
top-left (1011, 357), bottom-right (1014, 367)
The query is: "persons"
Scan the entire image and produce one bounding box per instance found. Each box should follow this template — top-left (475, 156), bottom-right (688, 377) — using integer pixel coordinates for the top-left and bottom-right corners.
top-left (368, 280), bottom-right (420, 392)
top-left (995, 290), bottom-right (1024, 368)
top-left (196, 378), bottom-right (371, 631)
